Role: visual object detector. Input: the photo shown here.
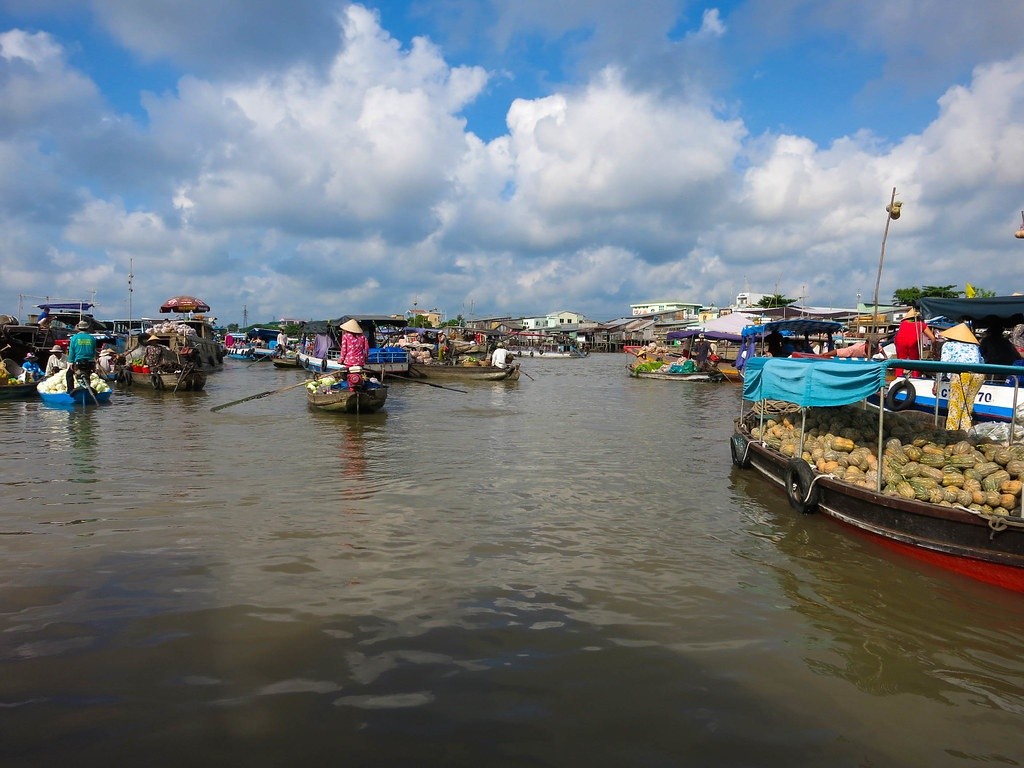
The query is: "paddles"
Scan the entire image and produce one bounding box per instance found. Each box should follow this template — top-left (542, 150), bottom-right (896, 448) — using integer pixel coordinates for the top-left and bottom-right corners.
top-left (245, 349), bottom-right (277, 368)
top-left (211, 367), bottom-right (348, 412)
top-left (363, 367), bottom-right (469, 395)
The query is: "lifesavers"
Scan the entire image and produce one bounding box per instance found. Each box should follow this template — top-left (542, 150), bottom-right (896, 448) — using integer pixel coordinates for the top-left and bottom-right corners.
top-left (729, 434), bottom-right (750, 469)
top-left (539, 350), bottom-right (543, 354)
top-left (197, 356), bottom-right (202, 367)
top-left (530, 352), bottom-right (533, 357)
top-left (886, 380), bottom-right (916, 411)
top-left (518, 351), bottom-right (521, 356)
top-left (210, 356), bottom-right (215, 365)
top-left (305, 358), bottom-right (309, 368)
top-left (322, 360), bottom-right (327, 370)
top-left (117, 369), bottom-right (132, 386)
top-left (151, 374), bottom-right (160, 389)
top-left (296, 354), bottom-right (299, 364)
top-left (784, 456), bottom-right (819, 514)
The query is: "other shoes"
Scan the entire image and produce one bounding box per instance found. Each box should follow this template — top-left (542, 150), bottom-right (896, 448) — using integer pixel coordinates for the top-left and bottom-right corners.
top-left (39, 325), bottom-right (47, 328)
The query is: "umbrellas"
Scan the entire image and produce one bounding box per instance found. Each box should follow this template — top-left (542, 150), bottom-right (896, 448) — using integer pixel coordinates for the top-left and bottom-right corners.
top-left (700, 312), bottom-right (770, 359)
top-left (159, 295), bottom-right (210, 348)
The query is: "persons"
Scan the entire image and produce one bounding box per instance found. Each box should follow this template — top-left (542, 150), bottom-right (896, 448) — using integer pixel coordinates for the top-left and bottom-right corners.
top-left (895, 309), bottom-right (936, 378)
top-left (980, 324), bottom-right (1024, 388)
top-left (97, 348), bottom-right (117, 373)
top-left (820, 336), bottom-right (888, 359)
top-left (464, 335), bottom-right (499, 345)
top-left (337, 319), bottom-right (369, 368)
top-left (66, 321), bottom-right (97, 394)
top-left (37, 308), bottom-right (57, 329)
top-left (692, 333), bottom-right (714, 373)
top-left (938, 323), bottom-right (986, 433)
top-left (45, 345), bottom-right (68, 376)
top-left (384, 335), bottom-right (407, 350)
top-left (141, 335), bottom-right (164, 372)
top-left (682, 337), bottom-right (695, 359)
top-left (417, 330), bottom-right (447, 361)
top-left (277, 329), bottom-right (289, 362)
top-left (228, 336), bottom-right (265, 348)
top-left (22, 352), bottom-right (45, 383)
top-left (492, 343), bottom-right (509, 370)
top-left (755, 330), bottom-right (815, 357)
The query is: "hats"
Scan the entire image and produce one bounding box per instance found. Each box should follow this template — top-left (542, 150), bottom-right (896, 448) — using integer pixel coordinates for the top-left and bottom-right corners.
top-left (107, 348), bottom-right (115, 352)
top-left (99, 350), bottom-right (110, 357)
top-left (49, 345), bottom-right (64, 353)
top-left (340, 319), bottom-right (364, 334)
top-left (938, 322), bottom-right (981, 346)
top-left (497, 343), bottom-right (504, 348)
top-left (900, 307), bottom-right (923, 322)
top-left (76, 321), bottom-right (90, 329)
top-left (24, 352), bottom-right (38, 362)
top-left (438, 330), bottom-right (444, 334)
top-left (698, 333), bottom-right (705, 339)
top-left (146, 335), bottom-right (160, 343)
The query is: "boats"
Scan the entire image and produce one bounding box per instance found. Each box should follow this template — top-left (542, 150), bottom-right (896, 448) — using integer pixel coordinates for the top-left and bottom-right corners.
top-left (307, 365), bottom-right (388, 414)
top-left (730, 318), bottom-right (1024, 594)
top-left (0, 318), bottom-right (591, 401)
top-left (624, 294), bottom-right (1024, 382)
top-left (36, 378), bottom-right (113, 405)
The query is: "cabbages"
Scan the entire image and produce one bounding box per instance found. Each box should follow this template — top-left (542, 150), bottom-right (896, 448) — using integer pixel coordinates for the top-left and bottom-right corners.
top-left (305, 378), bottom-right (336, 393)
top-left (37, 369), bottom-right (109, 393)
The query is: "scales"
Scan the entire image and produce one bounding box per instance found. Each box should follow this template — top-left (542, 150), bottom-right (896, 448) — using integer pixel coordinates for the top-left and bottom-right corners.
top-left (673, 338), bottom-right (682, 346)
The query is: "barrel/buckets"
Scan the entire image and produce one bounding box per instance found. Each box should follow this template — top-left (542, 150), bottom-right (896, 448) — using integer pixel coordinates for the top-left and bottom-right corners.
top-left (132, 365), bottom-right (150, 373)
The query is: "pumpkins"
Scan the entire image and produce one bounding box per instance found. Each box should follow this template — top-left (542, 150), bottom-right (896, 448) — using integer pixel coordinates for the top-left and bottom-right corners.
top-left (749, 406), bottom-right (1024, 517)
